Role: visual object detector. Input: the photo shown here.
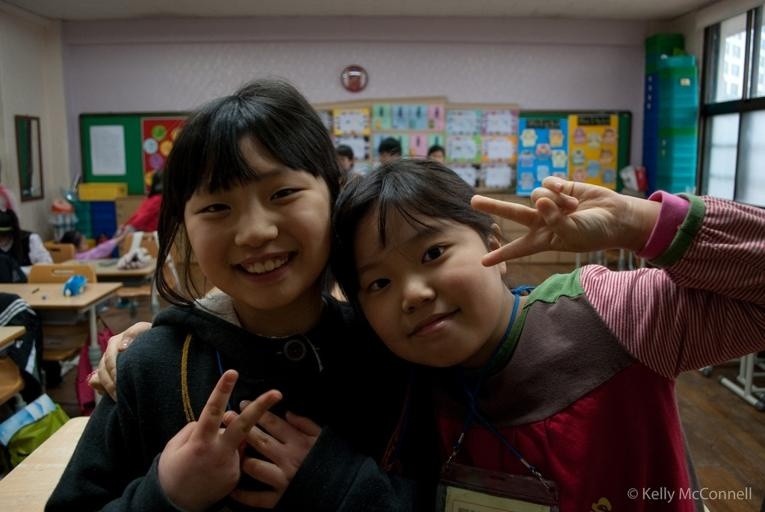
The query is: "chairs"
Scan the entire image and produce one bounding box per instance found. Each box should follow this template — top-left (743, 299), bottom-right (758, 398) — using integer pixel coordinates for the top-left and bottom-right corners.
top-left (0, 232), bottom-right (177, 405)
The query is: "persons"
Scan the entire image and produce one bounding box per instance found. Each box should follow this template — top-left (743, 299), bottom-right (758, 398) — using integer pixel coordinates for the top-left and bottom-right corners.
top-left (0, 208), bottom-right (54, 276)
top-left (428, 146), bottom-right (445, 163)
top-left (43, 80), bottom-right (447, 512)
top-left (336, 145), bottom-right (366, 191)
top-left (60, 168), bottom-right (171, 260)
top-left (330, 159), bottom-right (765, 512)
top-left (378, 139), bottom-right (401, 164)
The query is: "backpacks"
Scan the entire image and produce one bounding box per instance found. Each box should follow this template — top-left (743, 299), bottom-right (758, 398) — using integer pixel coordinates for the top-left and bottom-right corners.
top-left (8, 404), bottom-right (71, 468)
top-left (76, 315), bottom-right (116, 416)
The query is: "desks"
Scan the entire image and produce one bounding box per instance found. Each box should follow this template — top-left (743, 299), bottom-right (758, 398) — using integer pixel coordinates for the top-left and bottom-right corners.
top-left (0, 415), bottom-right (92, 511)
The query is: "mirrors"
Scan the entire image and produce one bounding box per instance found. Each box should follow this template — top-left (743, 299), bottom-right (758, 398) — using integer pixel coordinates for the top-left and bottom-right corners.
top-left (14, 114), bottom-right (44, 203)
